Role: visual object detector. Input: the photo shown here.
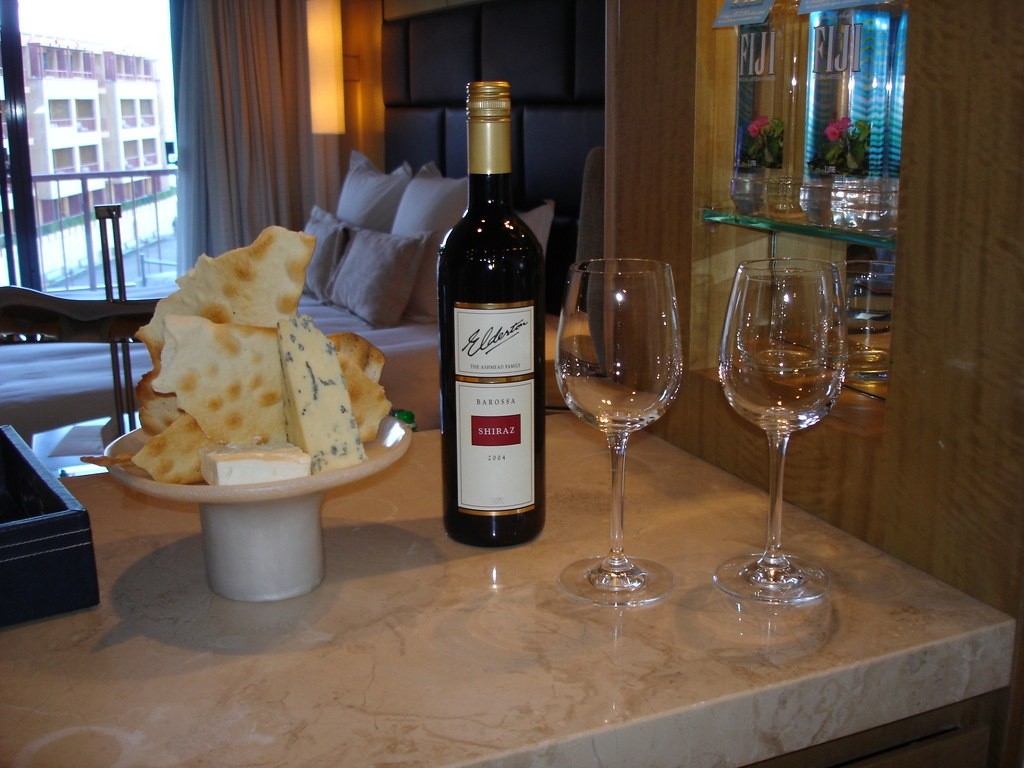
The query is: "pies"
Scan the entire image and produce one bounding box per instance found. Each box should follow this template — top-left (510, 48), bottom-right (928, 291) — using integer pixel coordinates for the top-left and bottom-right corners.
top-left (74, 223), bottom-right (392, 486)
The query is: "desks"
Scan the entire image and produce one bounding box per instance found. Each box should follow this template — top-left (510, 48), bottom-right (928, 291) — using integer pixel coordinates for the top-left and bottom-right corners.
top-left (0, 410), bottom-right (1017, 768)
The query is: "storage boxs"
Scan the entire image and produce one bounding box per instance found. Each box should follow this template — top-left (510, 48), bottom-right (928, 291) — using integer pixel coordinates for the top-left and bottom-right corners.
top-left (0, 423), bottom-right (102, 627)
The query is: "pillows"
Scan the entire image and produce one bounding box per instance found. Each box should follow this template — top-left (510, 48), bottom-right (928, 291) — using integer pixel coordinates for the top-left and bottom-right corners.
top-left (303, 205), bottom-right (351, 306)
top-left (323, 229), bottom-right (439, 328)
top-left (337, 149), bottom-right (414, 232)
top-left (390, 160), bottom-right (467, 321)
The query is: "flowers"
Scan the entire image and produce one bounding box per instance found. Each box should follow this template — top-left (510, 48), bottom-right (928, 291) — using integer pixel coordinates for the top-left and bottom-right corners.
top-left (748, 115), bottom-right (785, 169)
top-left (806, 117), bottom-right (871, 180)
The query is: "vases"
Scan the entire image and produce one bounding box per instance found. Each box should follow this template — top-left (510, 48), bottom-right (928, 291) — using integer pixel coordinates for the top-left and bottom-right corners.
top-left (734, 167), bottom-right (782, 216)
top-left (807, 173), bottom-right (866, 226)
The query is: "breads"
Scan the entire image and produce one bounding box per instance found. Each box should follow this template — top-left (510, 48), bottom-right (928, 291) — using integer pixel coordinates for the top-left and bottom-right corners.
top-left (276, 313), bottom-right (367, 477)
top-left (197, 442), bottom-right (312, 486)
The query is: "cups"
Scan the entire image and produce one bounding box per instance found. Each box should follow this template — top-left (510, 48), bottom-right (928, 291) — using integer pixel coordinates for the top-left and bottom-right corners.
top-left (844, 259), bottom-right (894, 385)
top-left (731, 175), bottom-right (899, 233)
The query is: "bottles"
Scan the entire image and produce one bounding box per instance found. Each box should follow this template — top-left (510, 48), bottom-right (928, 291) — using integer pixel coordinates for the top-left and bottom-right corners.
top-left (435, 80), bottom-right (548, 549)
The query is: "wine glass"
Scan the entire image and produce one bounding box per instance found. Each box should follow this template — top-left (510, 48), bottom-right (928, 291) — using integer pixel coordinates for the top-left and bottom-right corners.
top-left (553, 257), bottom-right (684, 607)
top-left (713, 257), bottom-right (848, 606)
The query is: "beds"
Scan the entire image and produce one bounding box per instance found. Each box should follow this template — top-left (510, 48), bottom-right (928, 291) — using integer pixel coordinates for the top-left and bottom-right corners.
top-left (0, 198), bottom-right (557, 444)
